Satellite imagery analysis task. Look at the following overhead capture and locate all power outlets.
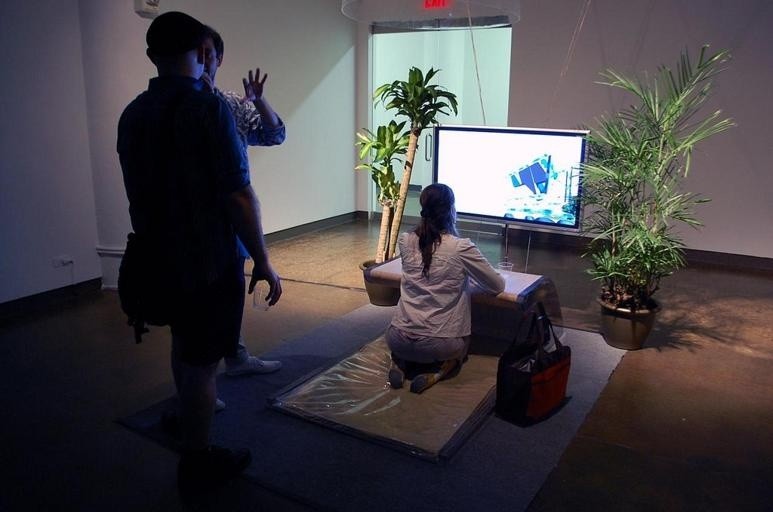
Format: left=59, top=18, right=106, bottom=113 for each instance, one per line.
left=52, top=254, right=72, bottom=267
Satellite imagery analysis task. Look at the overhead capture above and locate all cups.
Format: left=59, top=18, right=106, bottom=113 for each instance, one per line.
left=495, top=261, right=512, bottom=285
left=251, top=277, right=276, bottom=312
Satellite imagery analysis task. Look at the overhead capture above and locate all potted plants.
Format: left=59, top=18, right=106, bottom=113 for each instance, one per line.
left=578, top=42, right=741, bottom=351
left=359, top=63, right=460, bottom=306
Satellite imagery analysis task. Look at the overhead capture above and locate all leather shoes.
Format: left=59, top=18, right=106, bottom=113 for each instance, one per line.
left=388, top=351, right=406, bottom=389
left=409, top=359, right=449, bottom=394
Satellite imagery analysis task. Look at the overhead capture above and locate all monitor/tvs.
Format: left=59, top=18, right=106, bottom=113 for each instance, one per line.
left=432, top=124, right=591, bottom=235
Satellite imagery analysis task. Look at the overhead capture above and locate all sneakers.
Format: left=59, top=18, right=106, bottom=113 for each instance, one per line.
left=225, top=355, right=282, bottom=377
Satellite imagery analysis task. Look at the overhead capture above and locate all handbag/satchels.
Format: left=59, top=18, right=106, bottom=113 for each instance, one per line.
left=496, top=299, right=571, bottom=426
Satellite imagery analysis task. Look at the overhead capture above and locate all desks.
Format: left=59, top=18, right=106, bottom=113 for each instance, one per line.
left=366, top=252, right=563, bottom=356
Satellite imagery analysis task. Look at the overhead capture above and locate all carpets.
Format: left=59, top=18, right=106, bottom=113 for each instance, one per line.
left=108, top=291, right=626, bottom=512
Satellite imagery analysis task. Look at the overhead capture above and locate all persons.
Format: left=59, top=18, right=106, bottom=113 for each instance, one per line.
left=194, top=19, right=285, bottom=413
left=114, top=11, right=283, bottom=496
left=386, top=183, right=504, bottom=395
